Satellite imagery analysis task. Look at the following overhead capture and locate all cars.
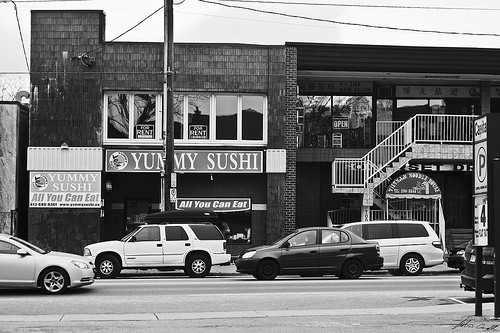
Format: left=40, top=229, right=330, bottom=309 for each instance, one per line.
left=0, top=232, right=95, bottom=295
left=235, top=226, right=385, bottom=281
left=447, top=241, right=469, bottom=273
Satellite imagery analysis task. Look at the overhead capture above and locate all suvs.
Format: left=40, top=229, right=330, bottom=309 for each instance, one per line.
left=83, top=222, right=232, bottom=278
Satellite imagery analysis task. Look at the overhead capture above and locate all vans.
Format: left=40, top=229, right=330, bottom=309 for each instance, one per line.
left=459, top=238, right=494, bottom=295
left=322, top=220, right=447, bottom=277
